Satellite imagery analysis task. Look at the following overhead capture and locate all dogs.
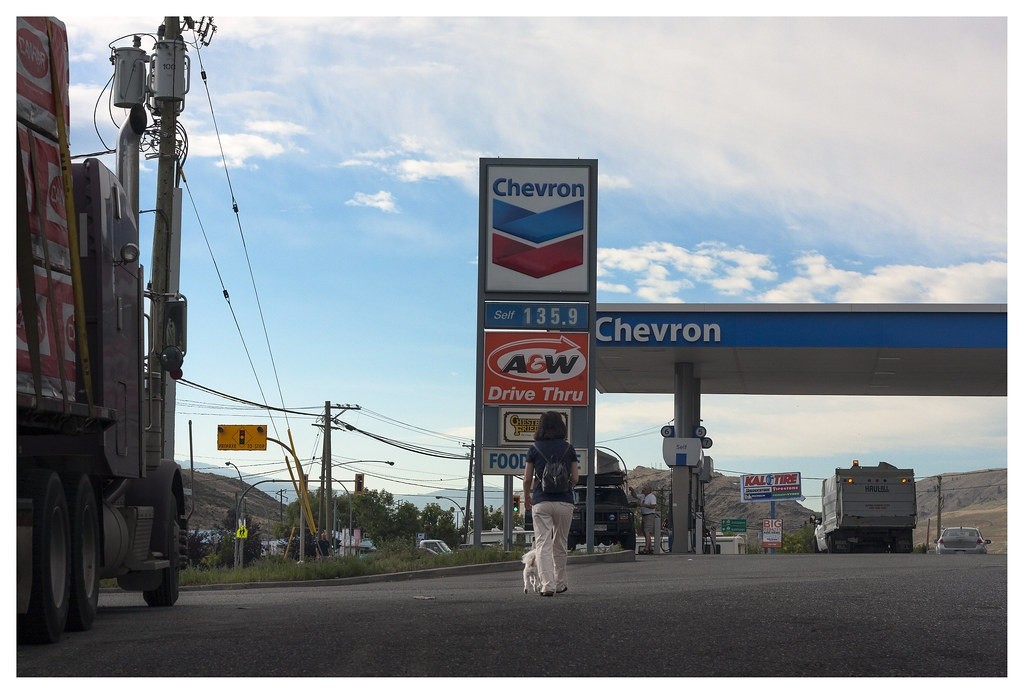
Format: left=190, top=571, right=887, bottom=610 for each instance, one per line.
left=521, top=540, right=543, bottom=595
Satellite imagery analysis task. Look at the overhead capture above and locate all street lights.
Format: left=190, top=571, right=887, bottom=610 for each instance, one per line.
left=435, top=495, right=466, bottom=519
left=222, top=460, right=247, bottom=510
left=318, top=473, right=353, bottom=537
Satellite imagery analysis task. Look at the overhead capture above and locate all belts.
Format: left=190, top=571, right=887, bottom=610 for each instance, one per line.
left=642, top=513, right=655, bottom=516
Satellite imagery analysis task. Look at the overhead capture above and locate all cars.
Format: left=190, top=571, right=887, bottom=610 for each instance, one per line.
left=420, top=539, right=451, bottom=553
left=935, top=525, right=991, bottom=555
left=566, top=484, right=639, bottom=553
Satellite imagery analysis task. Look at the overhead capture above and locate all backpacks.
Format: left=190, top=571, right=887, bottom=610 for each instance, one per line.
left=531, top=442, right=569, bottom=494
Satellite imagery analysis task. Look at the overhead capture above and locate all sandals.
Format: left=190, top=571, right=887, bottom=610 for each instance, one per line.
left=638, top=549, right=654, bottom=555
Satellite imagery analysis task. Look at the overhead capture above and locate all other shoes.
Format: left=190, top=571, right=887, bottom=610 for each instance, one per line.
left=555, top=587, right=568, bottom=593
left=540, top=591, right=554, bottom=597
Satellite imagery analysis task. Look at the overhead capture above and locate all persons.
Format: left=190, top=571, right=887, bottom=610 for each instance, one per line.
left=316, top=533, right=332, bottom=558
left=630, top=486, right=656, bottom=555
left=524, top=411, right=578, bottom=597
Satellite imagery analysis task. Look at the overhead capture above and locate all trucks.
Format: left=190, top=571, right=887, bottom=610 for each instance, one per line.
left=16, top=17, right=184, bottom=639
left=810, top=459, right=918, bottom=555
